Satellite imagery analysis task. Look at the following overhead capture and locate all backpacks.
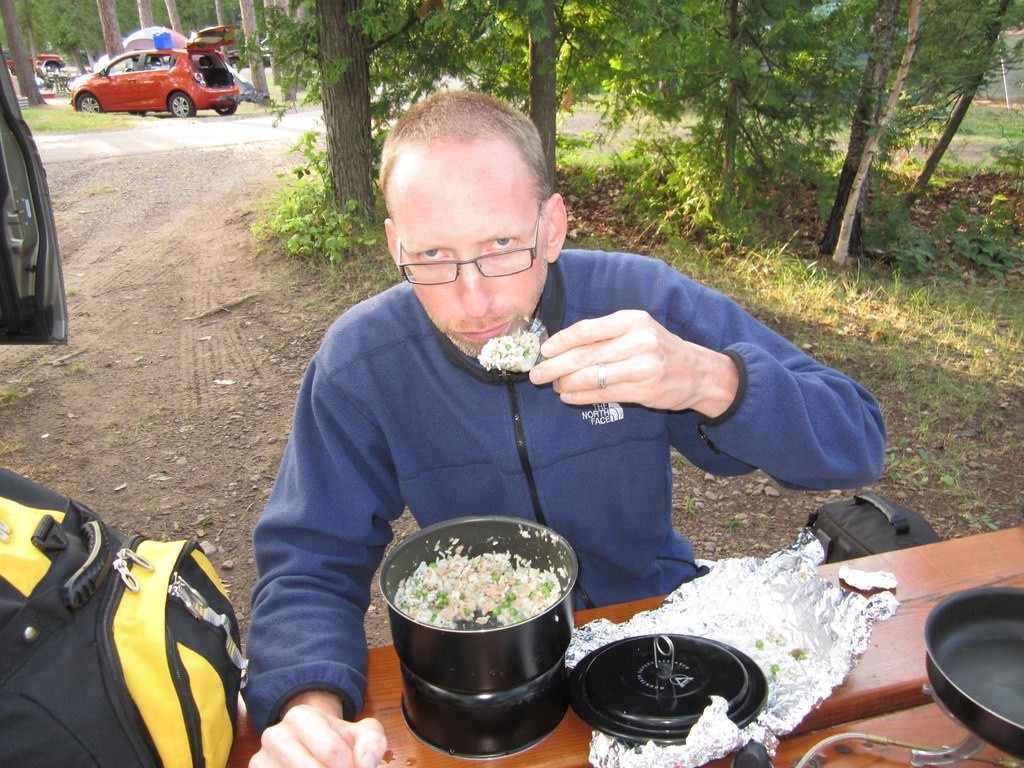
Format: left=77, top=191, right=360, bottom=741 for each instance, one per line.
left=2, top=469, right=242, bottom=765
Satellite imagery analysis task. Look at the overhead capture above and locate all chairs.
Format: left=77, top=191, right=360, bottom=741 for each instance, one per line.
left=198, top=57, right=210, bottom=69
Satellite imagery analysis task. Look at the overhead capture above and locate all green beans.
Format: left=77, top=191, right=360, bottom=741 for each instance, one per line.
left=399, top=561, right=555, bottom=627
left=498, top=333, right=530, bottom=357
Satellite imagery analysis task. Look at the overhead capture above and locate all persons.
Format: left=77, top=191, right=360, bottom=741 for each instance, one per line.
left=35, top=61, right=54, bottom=90
left=239, top=91, right=884, bottom=768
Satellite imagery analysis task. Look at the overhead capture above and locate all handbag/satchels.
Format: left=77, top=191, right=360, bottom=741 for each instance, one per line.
left=807, top=492, right=939, bottom=558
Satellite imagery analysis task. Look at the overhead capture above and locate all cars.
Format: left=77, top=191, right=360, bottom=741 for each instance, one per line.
left=70, top=23, right=242, bottom=121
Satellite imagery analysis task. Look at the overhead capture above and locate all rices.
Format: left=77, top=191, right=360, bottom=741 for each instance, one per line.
left=477, top=333, right=541, bottom=372
left=392, top=526, right=567, bottom=630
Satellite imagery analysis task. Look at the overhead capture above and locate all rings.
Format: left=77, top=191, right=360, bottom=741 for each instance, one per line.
left=598, top=362, right=608, bottom=389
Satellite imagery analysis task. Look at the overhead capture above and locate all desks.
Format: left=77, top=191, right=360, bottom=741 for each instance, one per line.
left=226, top=526, right=1024, bottom=768
left=47, top=75, right=72, bottom=98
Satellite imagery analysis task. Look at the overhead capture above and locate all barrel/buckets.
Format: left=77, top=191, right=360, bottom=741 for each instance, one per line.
left=153, top=31, right=172, bottom=50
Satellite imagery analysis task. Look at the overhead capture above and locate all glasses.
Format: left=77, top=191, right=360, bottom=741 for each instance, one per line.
left=397, top=206, right=542, bottom=285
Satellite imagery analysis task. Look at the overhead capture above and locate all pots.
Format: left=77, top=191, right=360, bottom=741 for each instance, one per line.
left=924, top=587, right=1024, bottom=759
left=378, top=514, right=578, bottom=761
